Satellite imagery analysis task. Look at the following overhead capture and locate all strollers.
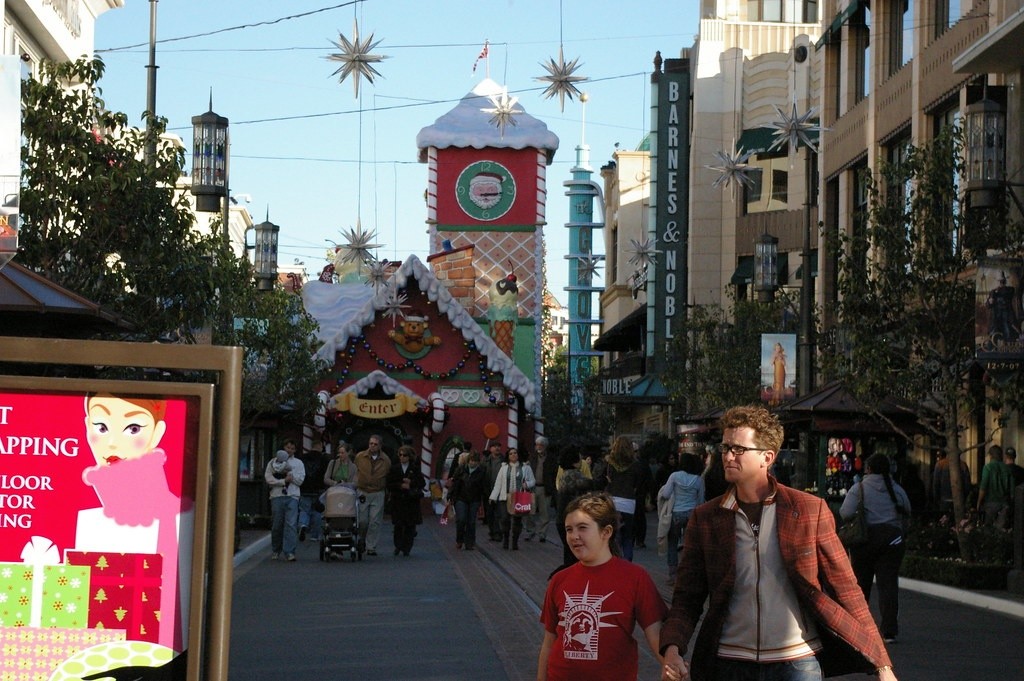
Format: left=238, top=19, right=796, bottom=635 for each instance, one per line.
left=318, top=486, right=362, bottom=563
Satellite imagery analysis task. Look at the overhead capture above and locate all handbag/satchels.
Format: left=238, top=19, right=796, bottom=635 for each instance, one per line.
left=853, top=523, right=906, bottom=565
left=409, top=486, right=425, bottom=500
left=506, top=486, right=538, bottom=517
left=440, top=505, right=456, bottom=526
left=315, top=491, right=326, bottom=513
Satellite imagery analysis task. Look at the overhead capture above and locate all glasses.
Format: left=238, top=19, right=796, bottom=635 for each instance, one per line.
left=718, top=443, right=768, bottom=455
left=399, top=453, right=408, bottom=457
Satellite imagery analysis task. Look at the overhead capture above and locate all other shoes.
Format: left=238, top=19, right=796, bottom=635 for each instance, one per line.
left=394, top=547, right=411, bottom=556
left=456, top=541, right=475, bottom=549
left=884, top=634, right=897, bottom=643
left=298, top=524, right=320, bottom=541
left=362, top=547, right=377, bottom=556
left=638, top=542, right=647, bottom=547
left=666, top=576, right=677, bottom=585
left=272, top=552, right=297, bottom=561
left=490, top=536, right=547, bottom=551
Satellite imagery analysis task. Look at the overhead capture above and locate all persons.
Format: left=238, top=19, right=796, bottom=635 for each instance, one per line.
left=659, top=403, right=901, bottom=681
left=264, top=434, right=425, bottom=562
left=429, top=436, right=790, bottom=586
left=537, top=490, right=690, bottom=681
left=929, top=450, right=972, bottom=511
left=825, top=438, right=862, bottom=503
left=978, top=445, right=1024, bottom=560
left=838, top=455, right=911, bottom=644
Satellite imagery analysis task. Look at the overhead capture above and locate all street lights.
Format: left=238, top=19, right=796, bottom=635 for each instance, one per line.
left=753, top=226, right=815, bottom=471
left=143, top=83, right=229, bottom=262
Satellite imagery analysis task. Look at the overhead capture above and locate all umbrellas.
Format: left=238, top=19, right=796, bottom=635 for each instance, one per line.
left=775, top=372, right=947, bottom=456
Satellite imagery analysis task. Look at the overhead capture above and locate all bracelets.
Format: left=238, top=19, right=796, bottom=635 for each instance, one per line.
left=874, top=666, right=891, bottom=675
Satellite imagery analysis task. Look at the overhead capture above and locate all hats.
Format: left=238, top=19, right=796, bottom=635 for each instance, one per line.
left=277, top=449, right=289, bottom=462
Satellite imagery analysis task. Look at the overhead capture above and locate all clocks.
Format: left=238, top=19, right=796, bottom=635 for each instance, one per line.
left=455, top=161, right=517, bottom=222
